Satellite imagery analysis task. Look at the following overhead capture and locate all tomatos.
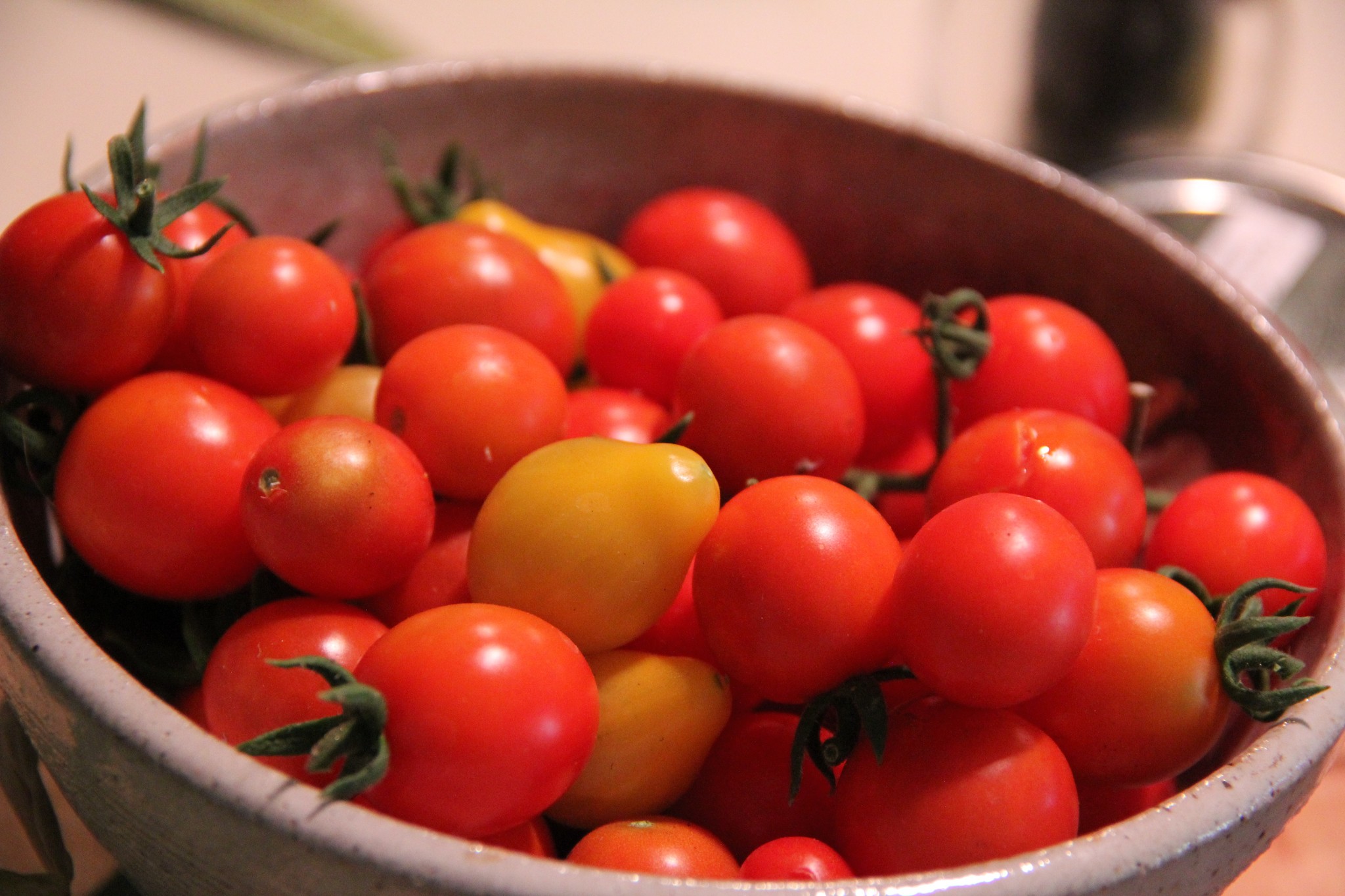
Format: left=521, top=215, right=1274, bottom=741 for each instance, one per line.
left=0, top=84, right=1331, bottom=886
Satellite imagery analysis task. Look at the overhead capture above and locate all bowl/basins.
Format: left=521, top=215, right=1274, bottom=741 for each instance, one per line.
left=0, top=55, right=1344, bottom=896
